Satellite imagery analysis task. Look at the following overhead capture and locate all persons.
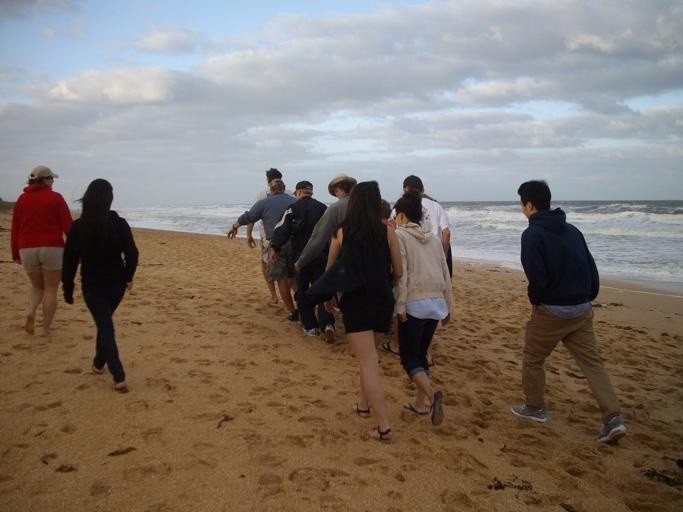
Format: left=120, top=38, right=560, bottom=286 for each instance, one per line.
left=61, top=179, right=139, bottom=393
left=294, top=181, right=403, bottom=443
left=229, top=169, right=358, bottom=342
left=512, top=179, right=628, bottom=443
left=382, top=174, right=451, bottom=424
left=11, top=165, right=74, bottom=336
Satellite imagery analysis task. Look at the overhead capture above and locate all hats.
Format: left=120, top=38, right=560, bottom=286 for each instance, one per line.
left=292, top=180, right=313, bottom=196
left=29, top=164, right=57, bottom=180
left=268, top=178, right=284, bottom=187
left=328, top=176, right=357, bottom=197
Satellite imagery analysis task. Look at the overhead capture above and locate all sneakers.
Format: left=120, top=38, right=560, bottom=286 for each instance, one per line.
left=289, top=309, right=337, bottom=343
left=511, top=404, right=547, bottom=423
left=597, top=416, right=626, bottom=443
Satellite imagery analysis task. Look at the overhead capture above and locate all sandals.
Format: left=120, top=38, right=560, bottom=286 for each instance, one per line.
left=353, top=402, right=371, bottom=418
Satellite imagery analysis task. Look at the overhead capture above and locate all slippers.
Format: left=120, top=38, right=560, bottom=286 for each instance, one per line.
left=431, top=390, right=445, bottom=425
left=381, top=339, right=399, bottom=355
left=368, top=424, right=394, bottom=444
left=404, top=401, right=430, bottom=416
